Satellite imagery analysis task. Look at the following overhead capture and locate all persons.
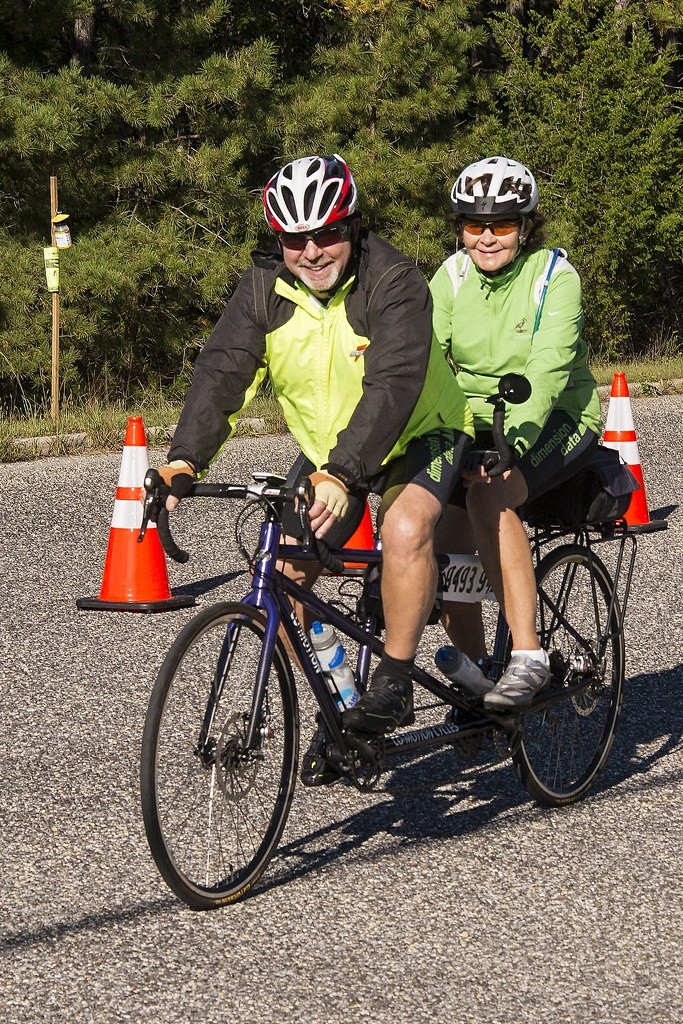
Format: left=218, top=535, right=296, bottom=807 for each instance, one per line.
left=141, top=154, right=476, bottom=787
left=427, top=157, right=603, bottom=750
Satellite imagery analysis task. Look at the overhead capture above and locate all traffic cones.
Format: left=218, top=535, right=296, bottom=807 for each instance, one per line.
left=76, top=415, right=196, bottom=614
left=326, top=500, right=376, bottom=575
left=592, top=371, right=669, bottom=533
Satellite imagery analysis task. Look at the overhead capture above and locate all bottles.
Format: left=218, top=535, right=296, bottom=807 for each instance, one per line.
left=310, top=621, right=361, bottom=713
left=434, top=646, right=493, bottom=695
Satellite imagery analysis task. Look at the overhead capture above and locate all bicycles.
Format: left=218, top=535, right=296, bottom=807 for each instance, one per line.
left=136, top=372, right=640, bottom=912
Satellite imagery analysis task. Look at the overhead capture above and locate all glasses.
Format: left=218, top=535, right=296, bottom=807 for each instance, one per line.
left=460, top=218, right=521, bottom=236
left=273, top=225, right=347, bottom=250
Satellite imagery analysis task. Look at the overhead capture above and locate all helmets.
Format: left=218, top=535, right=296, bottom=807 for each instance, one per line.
left=449, top=156, right=539, bottom=214
left=263, top=153, right=357, bottom=233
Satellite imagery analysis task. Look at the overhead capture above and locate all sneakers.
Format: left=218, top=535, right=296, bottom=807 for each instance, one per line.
left=483, top=649, right=554, bottom=708
left=342, top=670, right=416, bottom=732
left=300, top=711, right=346, bottom=787
